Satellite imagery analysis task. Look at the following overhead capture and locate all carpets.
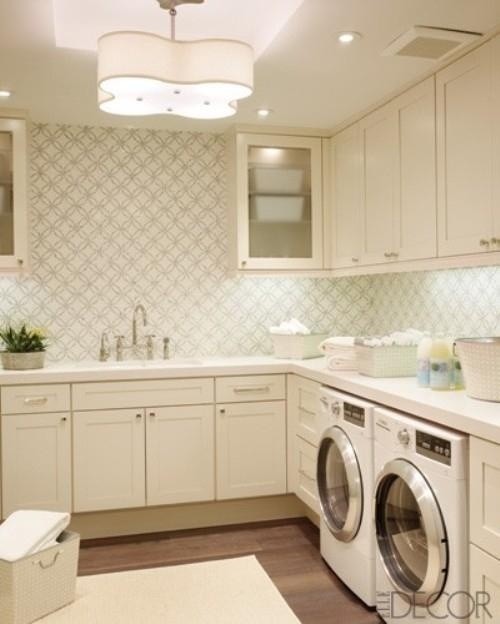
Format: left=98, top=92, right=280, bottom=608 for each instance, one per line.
left=32, top=554, right=302, bottom=624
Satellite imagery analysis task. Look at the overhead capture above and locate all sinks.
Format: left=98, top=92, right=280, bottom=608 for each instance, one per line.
left=86, top=357, right=205, bottom=370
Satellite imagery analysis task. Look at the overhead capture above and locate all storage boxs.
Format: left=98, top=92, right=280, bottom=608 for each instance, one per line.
left=0, top=509, right=81, bottom=624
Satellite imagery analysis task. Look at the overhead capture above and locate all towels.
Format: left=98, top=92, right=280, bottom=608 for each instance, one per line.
left=320, top=337, right=355, bottom=354
left=327, top=355, right=358, bottom=371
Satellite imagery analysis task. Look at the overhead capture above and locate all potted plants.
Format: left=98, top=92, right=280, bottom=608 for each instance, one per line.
left=0, top=321, right=53, bottom=369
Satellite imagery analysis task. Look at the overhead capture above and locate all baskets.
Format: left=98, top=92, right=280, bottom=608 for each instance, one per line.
left=319, top=336, right=354, bottom=369
left=272, top=333, right=326, bottom=358
left=456, top=338, right=499, bottom=402
left=355, top=337, right=415, bottom=378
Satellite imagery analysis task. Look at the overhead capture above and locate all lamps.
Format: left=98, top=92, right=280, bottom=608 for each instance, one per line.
left=95, top=0, right=254, bottom=120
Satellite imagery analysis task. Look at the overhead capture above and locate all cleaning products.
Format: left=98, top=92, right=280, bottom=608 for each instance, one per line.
left=418, top=326, right=468, bottom=392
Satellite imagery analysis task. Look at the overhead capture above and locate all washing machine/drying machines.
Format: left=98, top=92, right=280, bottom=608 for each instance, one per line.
left=373, top=406, right=470, bottom=624
left=315, top=386, right=382, bottom=608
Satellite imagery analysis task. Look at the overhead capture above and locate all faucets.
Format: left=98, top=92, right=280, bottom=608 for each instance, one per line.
left=133, top=303, right=148, bottom=345
left=100, top=332, right=111, bottom=361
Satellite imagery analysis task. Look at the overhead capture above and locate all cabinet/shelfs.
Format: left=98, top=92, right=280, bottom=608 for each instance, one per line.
left=0, top=110, right=28, bottom=275
left=361, top=73, right=436, bottom=276
left=0, top=382, right=72, bottom=522
left=228, top=123, right=322, bottom=276
left=436, top=34, right=500, bottom=269
left=288, top=374, right=322, bottom=517
left=466, top=435, right=500, bottom=624
left=214, top=374, right=287, bottom=502
left=330, top=96, right=364, bottom=277
left=72, top=377, right=217, bottom=513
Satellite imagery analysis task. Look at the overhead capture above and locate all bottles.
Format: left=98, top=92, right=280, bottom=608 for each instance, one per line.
left=416, top=331, right=465, bottom=391
